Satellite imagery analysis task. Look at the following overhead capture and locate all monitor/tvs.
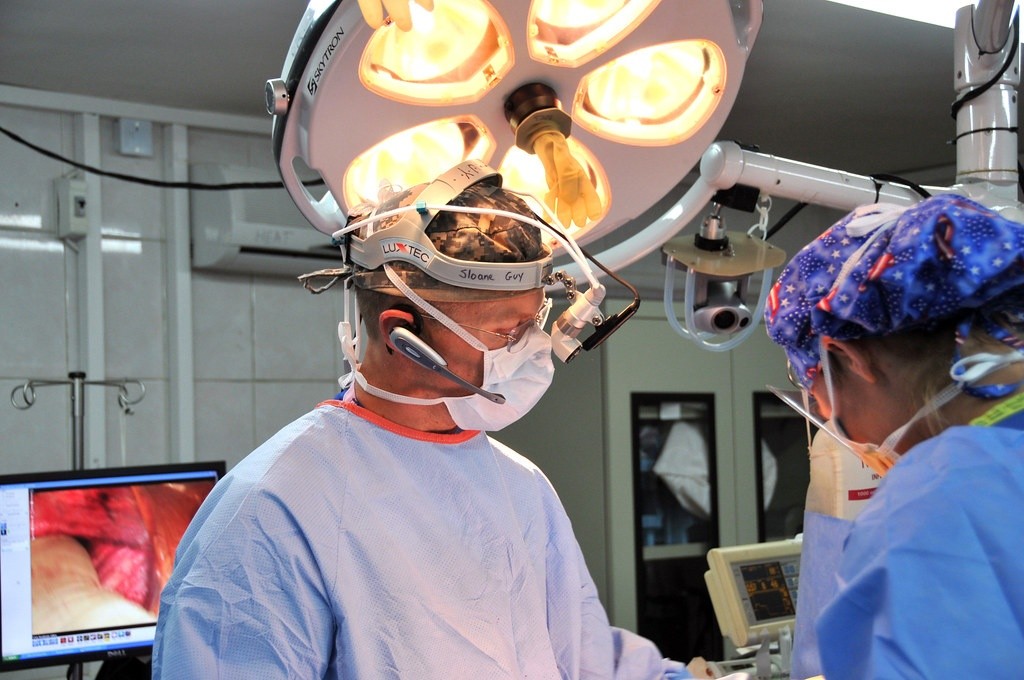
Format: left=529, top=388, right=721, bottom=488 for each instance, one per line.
left=0, top=460, right=229, bottom=676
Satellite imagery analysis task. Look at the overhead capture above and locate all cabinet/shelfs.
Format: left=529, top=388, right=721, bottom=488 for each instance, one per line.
left=599, top=299, right=819, bottom=664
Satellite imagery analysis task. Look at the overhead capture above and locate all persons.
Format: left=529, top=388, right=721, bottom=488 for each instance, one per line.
left=763, top=192, right=1024, bottom=680
left=150, top=161, right=721, bottom=680
left=30, top=534, right=156, bottom=635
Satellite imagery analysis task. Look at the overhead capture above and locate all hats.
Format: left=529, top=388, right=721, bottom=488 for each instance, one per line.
left=815, top=193, right=1024, bottom=346
left=765, top=204, right=908, bottom=380
left=343, top=180, right=547, bottom=302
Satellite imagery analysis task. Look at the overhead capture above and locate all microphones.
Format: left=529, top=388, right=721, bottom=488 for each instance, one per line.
left=389, top=324, right=506, bottom=411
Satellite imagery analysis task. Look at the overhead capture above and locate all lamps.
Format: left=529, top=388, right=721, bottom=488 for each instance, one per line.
left=264, top=0, right=764, bottom=294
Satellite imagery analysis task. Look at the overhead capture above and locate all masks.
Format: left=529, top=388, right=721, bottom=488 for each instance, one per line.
left=413, top=296, right=555, bottom=431
left=806, top=353, right=939, bottom=522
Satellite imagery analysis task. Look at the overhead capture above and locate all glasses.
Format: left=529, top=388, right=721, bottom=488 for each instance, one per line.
left=783, top=358, right=826, bottom=390
left=421, top=298, right=554, bottom=355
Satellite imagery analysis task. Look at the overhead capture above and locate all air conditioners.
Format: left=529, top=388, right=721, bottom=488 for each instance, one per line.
left=187, top=159, right=347, bottom=281
